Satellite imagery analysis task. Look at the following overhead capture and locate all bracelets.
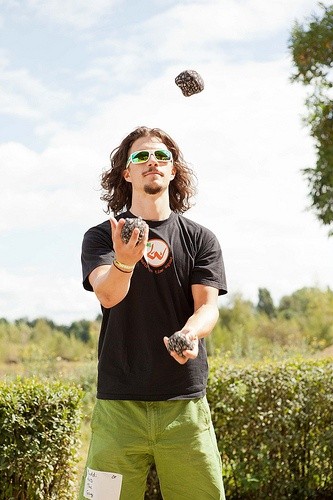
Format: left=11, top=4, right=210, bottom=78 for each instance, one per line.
left=112, top=259, right=136, bottom=274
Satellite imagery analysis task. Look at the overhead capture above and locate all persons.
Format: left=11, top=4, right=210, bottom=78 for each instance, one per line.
left=77, top=127, right=228, bottom=500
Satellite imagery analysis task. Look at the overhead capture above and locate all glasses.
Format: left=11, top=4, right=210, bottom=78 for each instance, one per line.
left=126, top=150, right=173, bottom=168
